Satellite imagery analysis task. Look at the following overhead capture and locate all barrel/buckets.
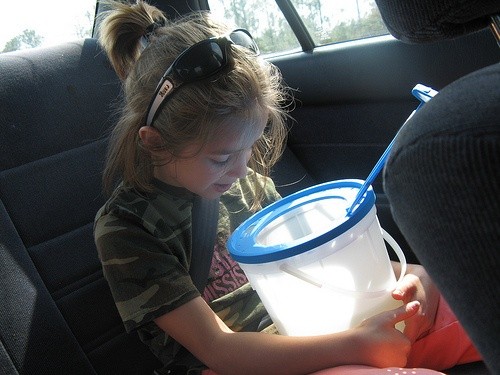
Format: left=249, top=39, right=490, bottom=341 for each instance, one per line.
left=226, top=180, right=408, bottom=338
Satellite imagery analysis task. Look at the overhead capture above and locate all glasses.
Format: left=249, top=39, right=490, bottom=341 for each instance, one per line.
left=145, top=28, right=260, bottom=127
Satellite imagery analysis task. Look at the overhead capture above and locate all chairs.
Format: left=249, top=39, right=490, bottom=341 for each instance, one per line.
left=0, top=39, right=190, bottom=375
left=375, top=0, right=500, bottom=375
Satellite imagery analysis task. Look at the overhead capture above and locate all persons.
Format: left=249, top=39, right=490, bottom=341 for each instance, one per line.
left=90, top=1, right=482, bottom=374
